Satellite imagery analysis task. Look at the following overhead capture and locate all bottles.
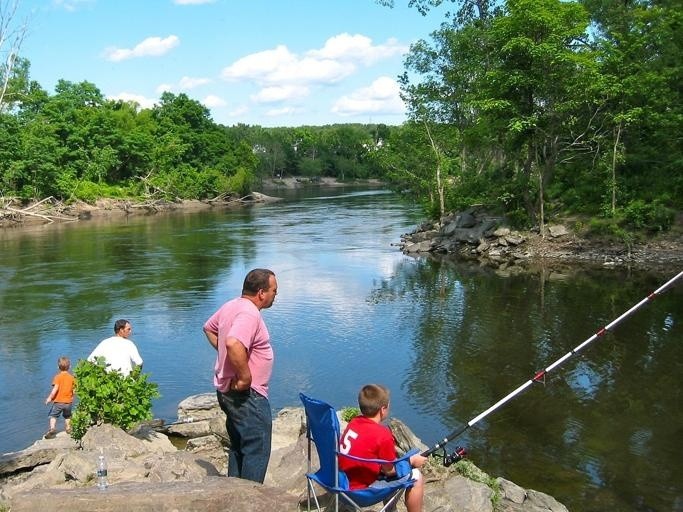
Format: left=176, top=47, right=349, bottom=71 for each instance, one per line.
left=97, top=455, right=109, bottom=493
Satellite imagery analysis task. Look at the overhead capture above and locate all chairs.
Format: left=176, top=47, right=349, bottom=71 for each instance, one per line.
left=299, top=393, right=417, bottom=512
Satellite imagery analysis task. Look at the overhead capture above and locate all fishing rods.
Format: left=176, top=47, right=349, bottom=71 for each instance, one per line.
left=420, top=270, right=683, bottom=468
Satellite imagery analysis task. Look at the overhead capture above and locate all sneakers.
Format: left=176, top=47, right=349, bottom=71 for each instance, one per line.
left=45, top=429, right=57, bottom=439
left=64, top=425, right=72, bottom=432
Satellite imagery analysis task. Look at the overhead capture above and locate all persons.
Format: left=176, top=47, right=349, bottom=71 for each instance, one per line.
left=87, top=319, right=143, bottom=379
left=203, top=268, right=277, bottom=484
left=45, top=356, right=76, bottom=439
left=338, top=384, right=428, bottom=512
